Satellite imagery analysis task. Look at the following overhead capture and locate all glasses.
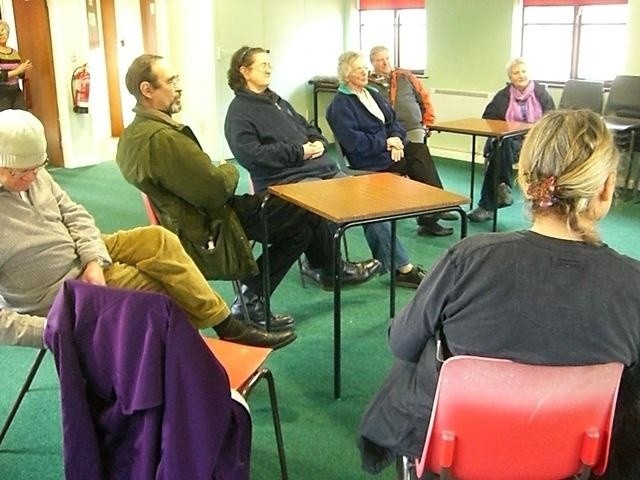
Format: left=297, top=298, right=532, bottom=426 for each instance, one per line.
left=6, top=159, right=50, bottom=178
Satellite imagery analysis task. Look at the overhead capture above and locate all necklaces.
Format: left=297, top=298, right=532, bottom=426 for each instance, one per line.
left=0, top=47, right=12, bottom=58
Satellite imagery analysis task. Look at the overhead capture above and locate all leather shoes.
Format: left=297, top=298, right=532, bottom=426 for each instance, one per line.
left=416, top=209, right=458, bottom=239
left=219, top=326, right=297, bottom=351
left=229, top=293, right=296, bottom=325
left=299, top=258, right=384, bottom=295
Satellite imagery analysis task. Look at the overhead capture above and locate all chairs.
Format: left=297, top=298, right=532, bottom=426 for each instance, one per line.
left=555, top=78, right=604, bottom=120
left=56, top=276, right=294, bottom=480
left=140, top=186, right=251, bottom=326
left=391, top=355, right=626, bottom=480
left=247, top=173, right=350, bottom=292
left=484, top=80, right=548, bottom=174
left=323, top=101, right=409, bottom=178
left=594, top=76, right=640, bottom=205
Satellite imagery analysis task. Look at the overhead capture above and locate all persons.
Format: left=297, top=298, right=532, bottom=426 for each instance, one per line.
left=1, top=20, right=36, bottom=112
left=223, top=44, right=428, bottom=289
left=325, top=50, right=460, bottom=237
left=0, top=108, right=298, bottom=351
left=465, top=57, right=557, bottom=223
left=355, top=103, right=640, bottom=480
left=365, top=45, right=437, bottom=146
left=115, top=53, right=384, bottom=328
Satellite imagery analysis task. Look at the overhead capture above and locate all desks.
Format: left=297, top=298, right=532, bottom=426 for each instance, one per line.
left=417, top=115, right=540, bottom=239
left=308, top=70, right=388, bottom=138
left=248, top=168, right=477, bottom=404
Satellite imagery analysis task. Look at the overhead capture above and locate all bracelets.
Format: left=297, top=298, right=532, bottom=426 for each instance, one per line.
left=96, top=256, right=105, bottom=267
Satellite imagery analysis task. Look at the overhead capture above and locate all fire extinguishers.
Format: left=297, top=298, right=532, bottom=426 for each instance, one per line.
left=71, top=63, right=92, bottom=115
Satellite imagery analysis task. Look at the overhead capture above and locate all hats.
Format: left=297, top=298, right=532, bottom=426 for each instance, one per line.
left=0, top=108, right=48, bottom=170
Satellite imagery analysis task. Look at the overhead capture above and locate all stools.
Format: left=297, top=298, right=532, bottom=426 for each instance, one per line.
left=0, top=341, right=50, bottom=456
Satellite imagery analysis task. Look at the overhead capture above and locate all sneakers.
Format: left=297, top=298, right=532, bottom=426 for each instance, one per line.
left=496, top=183, right=514, bottom=208
left=392, top=266, right=433, bottom=289
left=466, top=206, right=494, bottom=222
